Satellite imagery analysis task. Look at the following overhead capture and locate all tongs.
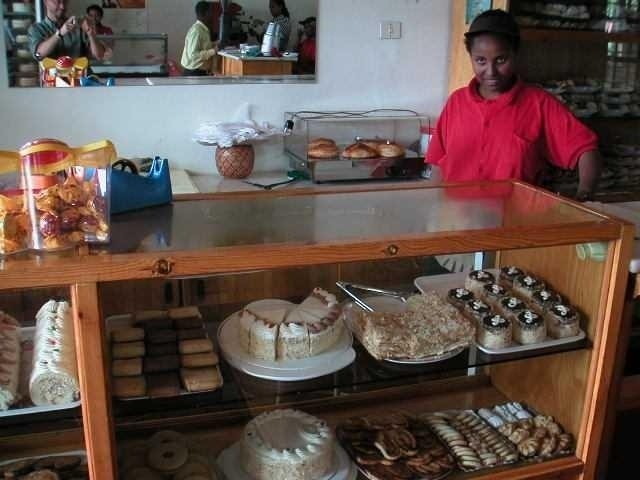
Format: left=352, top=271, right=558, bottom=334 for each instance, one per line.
left=335, top=278, right=411, bottom=313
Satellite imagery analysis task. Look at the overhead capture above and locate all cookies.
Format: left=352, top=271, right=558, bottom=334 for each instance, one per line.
left=338, top=401, right=574, bottom=480
left=0, top=174, right=109, bottom=256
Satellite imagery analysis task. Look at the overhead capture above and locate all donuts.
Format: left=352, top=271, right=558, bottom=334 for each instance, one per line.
left=118, top=431, right=216, bottom=478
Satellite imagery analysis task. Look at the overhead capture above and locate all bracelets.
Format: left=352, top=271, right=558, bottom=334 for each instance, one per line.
left=55, top=27, right=65, bottom=39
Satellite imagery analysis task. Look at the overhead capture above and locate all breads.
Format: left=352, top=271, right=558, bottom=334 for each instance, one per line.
left=110, top=307, right=220, bottom=395
left=0, top=264, right=79, bottom=480
left=307, top=138, right=405, bottom=159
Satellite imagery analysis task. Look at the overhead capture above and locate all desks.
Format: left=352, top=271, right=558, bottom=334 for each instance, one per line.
left=216, top=46, right=299, bottom=76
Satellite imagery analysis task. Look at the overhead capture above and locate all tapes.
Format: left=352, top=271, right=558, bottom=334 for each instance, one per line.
left=87, top=74, right=107, bottom=83
left=112, top=158, right=153, bottom=175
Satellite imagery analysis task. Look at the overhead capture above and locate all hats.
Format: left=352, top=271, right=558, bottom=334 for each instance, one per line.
left=298, top=17, right=315, bottom=25
left=463, top=10, right=521, bottom=43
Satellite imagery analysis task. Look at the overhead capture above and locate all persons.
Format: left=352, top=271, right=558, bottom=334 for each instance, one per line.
left=295, top=16, right=317, bottom=74
left=86, top=4, right=115, bottom=60
left=246, top=0, right=291, bottom=49
left=180, top=0, right=232, bottom=77
left=424, top=7, right=601, bottom=203
left=26, top=0, right=106, bottom=65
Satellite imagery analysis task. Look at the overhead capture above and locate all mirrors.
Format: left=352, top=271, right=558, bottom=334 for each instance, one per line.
left=1, top=0, right=317, bottom=88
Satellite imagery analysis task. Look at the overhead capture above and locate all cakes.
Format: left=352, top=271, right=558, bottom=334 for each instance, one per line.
left=238, top=288, right=342, bottom=360
left=241, top=409, right=335, bottom=478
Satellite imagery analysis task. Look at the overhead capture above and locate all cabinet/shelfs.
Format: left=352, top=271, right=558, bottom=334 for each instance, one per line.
left=1, top=178, right=635, bottom=480
left=444, top=0, right=639, bottom=204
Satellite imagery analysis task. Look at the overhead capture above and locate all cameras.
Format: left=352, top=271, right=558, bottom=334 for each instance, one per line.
left=71, top=16, right=85, bottom=26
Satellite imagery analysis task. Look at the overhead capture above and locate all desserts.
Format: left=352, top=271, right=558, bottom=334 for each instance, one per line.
left=349, top=291, right=474, bottom=360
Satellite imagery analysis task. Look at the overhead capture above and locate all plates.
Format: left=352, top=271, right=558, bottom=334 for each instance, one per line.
left=216, top=296, right=356, bottom=384
left=215, top=433, right=359, bottom=480
left=343, top=293, right=472, bottom=366
